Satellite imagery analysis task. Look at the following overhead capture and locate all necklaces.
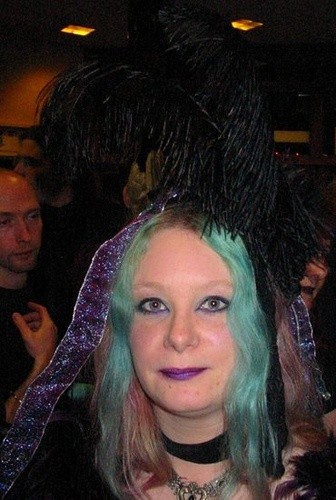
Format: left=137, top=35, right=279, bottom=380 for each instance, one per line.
left=160, top=428, right=229, bottom=464
left=170, top=470, right=232, bottom=500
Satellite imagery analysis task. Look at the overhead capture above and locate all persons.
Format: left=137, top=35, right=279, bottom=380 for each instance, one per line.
left=13, top=126, right=120, bottom=277
left=0, top=168, right=78, bottom=439
left=300, top=241, right=336, bottom=436
left=0, top=192, right=336, bottom=500
